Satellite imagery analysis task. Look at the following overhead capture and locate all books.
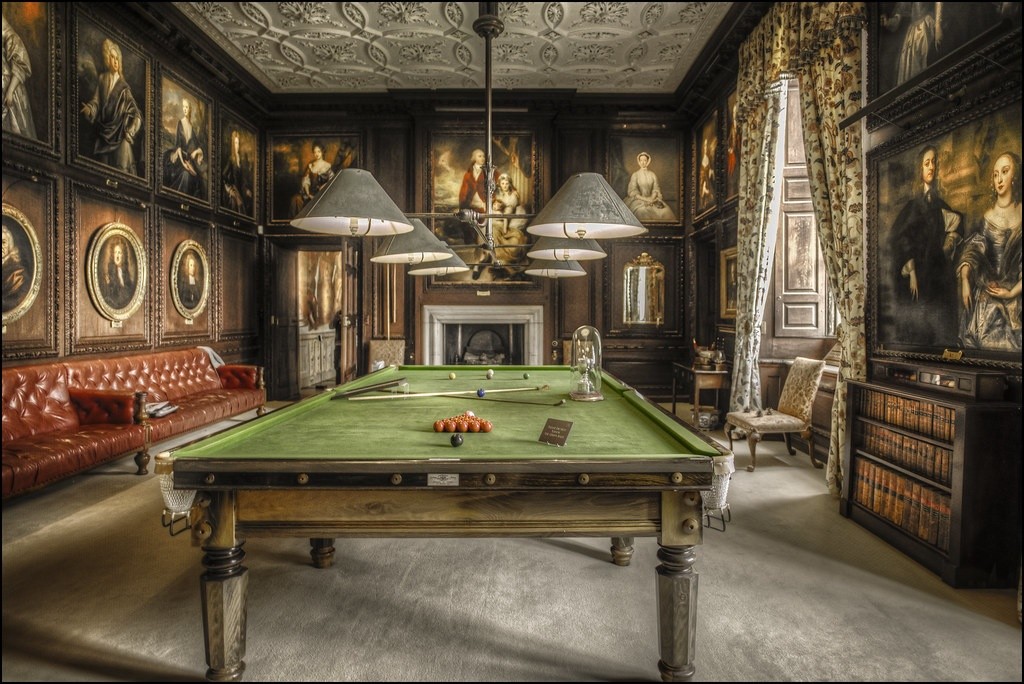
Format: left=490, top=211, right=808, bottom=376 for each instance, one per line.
left=852, top=387, right=956, bottom=555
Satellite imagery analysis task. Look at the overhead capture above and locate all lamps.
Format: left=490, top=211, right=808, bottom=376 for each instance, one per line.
left=290, top=7, right=649, bottom=278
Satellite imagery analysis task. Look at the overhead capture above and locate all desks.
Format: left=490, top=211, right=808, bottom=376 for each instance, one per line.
left=154, top=363, right=735, bottom=682
left=672, top=361, right=729, bottom=427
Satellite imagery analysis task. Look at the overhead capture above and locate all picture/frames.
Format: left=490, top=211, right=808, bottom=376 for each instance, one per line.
left=720, top=247, right=737, bottom=318
left=866, top=90, right=1023, bottom=375
left=0, top=0, right=265, bottom=362
left=601, top=236, right=689, bottom=340
left=265, top=128, right=364, bottom=228
left=691, top=79, right=742, bottom=226
left=423, top=129, right=544, bottom=292
left=604, top=128, right=685, bottom=228
left=866, top=2, right=1022, bottom=134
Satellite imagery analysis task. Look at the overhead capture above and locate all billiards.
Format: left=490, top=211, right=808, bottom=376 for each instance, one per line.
left=486, top=369, right=494, bottom=379
left=433, top=411, right=492, bottom=449
left=523, top=373, right=529, bottom=379
left=477, top=388, right=485, bottom=397
left=448, top=372, right=457, bottom=380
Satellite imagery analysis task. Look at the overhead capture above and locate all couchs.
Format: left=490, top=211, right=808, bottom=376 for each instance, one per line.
left=2, top=346, right=266, bottom=504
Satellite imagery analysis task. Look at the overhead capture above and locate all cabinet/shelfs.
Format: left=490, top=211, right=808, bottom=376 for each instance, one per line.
left=840, top=379, right=1024, bottom=588
left=299, top=329, right=336, bottom=387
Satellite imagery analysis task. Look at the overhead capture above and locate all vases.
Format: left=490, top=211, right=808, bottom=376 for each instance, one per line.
left=689, top=407, right=720, bottom=431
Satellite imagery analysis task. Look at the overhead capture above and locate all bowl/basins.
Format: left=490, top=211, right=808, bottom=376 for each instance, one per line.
left=690, top=408, right=721, bottom=432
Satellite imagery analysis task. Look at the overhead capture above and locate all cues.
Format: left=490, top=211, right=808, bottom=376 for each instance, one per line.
left=347, top=388, right=539, bottom=401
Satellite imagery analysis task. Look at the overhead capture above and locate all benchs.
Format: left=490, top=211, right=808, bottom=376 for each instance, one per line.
left=724, top=356, right=826, bottom=472
